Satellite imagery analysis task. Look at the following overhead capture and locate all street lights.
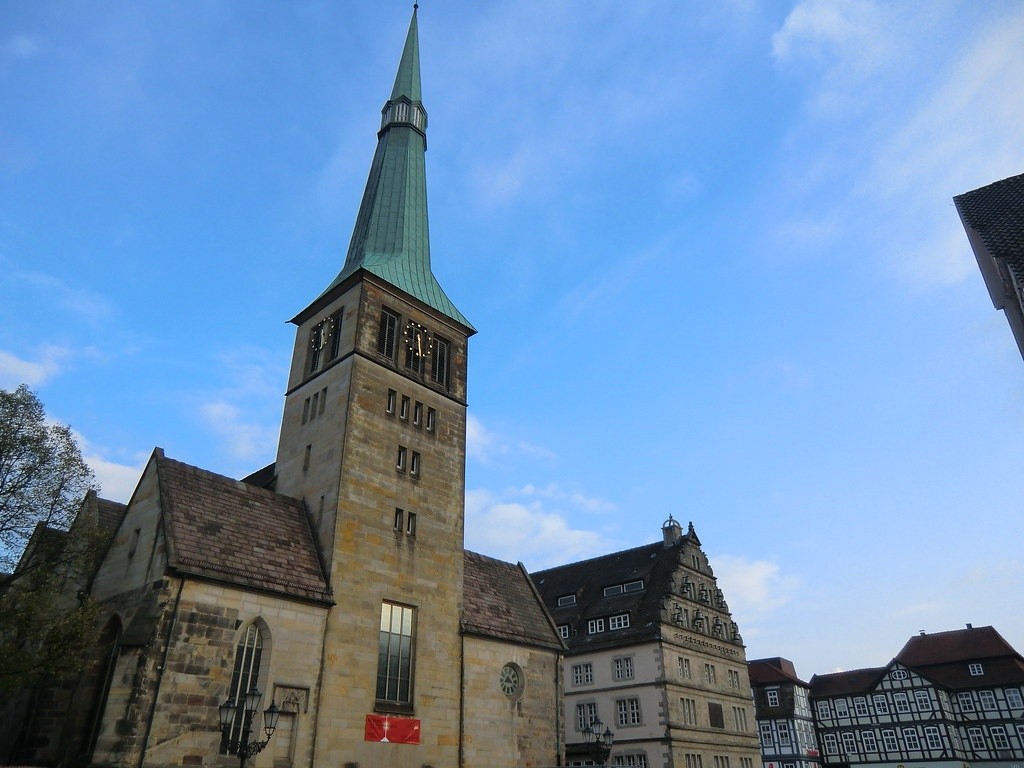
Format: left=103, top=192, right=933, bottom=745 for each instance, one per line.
left=218, top=682, right=281, bottom=767
left=581, top=718, right=614, bottom=767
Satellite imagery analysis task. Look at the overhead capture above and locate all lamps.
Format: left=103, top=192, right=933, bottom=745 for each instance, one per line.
left=219, top=682, right=280, bottom=768
left=582, top=716, right=614, bottom=766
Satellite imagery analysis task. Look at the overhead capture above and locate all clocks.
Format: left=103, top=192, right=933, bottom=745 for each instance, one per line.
left=312, top=317, right=335, bottom=352
left=404, top=322, right=432, bottom=358
